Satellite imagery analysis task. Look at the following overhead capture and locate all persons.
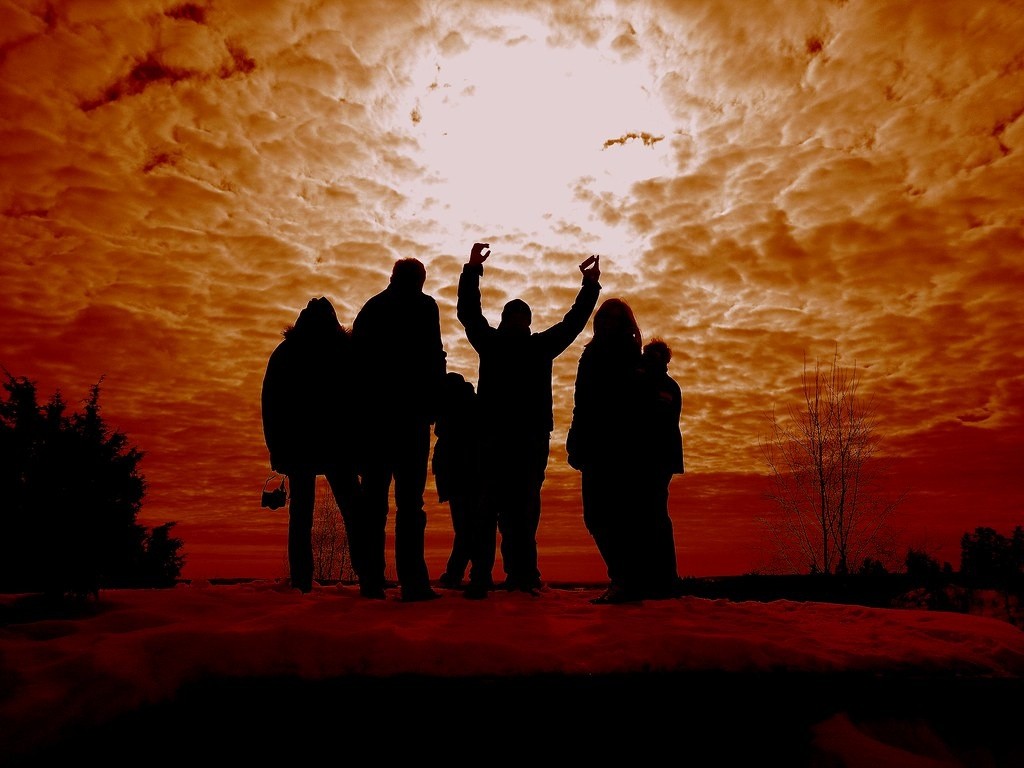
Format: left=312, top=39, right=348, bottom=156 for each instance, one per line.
left=261, top=244, right=601, bottom=601
left=567, top=299, right=684, bottom=603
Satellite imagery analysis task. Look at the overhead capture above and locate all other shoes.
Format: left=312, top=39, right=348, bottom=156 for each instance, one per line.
left=588, top=578, right=642, bottom=604
left=642, top=579, right=680, bottom=600
left=496, top=574, right=542, bottom=592
left=360, top=583, right=387, bottom=600
left=460, top=579, right=496, bottom=592
left=271, top=578, right=313, bottom=593
left=435, top=571, right=462, bottom=589
left=401, top=583, right=444, bottom=603
left=463, top=583, right=488, bottom=600
